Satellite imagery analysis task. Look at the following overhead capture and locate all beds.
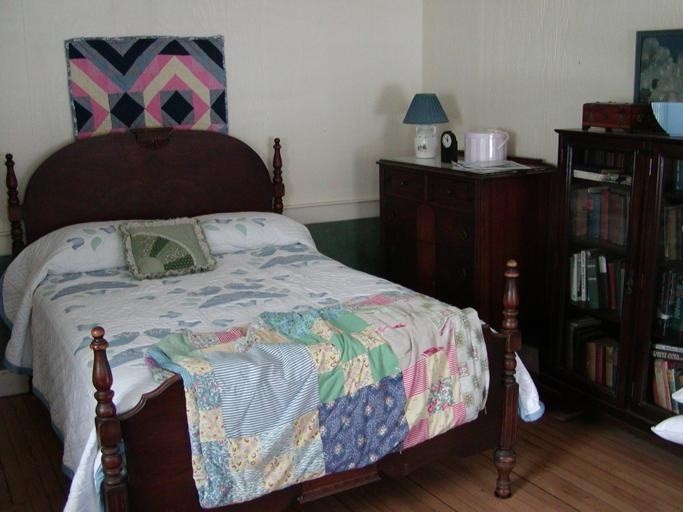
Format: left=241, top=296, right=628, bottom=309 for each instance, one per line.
left=0, top=128, right=546, bottom=512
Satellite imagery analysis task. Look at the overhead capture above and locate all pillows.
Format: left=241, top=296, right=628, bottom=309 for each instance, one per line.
left=118, top=217, right=217, bottom=280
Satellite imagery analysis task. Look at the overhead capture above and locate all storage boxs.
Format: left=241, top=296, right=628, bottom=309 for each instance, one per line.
left=580, top=102, right=634, bottom=134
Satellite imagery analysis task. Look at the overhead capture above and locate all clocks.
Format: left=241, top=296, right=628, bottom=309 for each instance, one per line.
left=441, top=133, right=456, bottom=160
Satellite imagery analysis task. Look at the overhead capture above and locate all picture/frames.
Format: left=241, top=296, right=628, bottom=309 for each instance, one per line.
left=634, top=30, right=683, bottom=108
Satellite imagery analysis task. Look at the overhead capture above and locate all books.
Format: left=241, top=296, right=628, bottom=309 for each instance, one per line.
left=561, top=147, right=683, bottom=414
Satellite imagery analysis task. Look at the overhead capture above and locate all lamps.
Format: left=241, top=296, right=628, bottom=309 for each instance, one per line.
left=402, top=92, right=451, bottom=157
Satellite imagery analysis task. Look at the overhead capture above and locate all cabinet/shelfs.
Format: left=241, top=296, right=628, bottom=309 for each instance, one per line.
left=553, top=125, right=682, bottom=455
left=372, top=152, right=558, bottom=414
